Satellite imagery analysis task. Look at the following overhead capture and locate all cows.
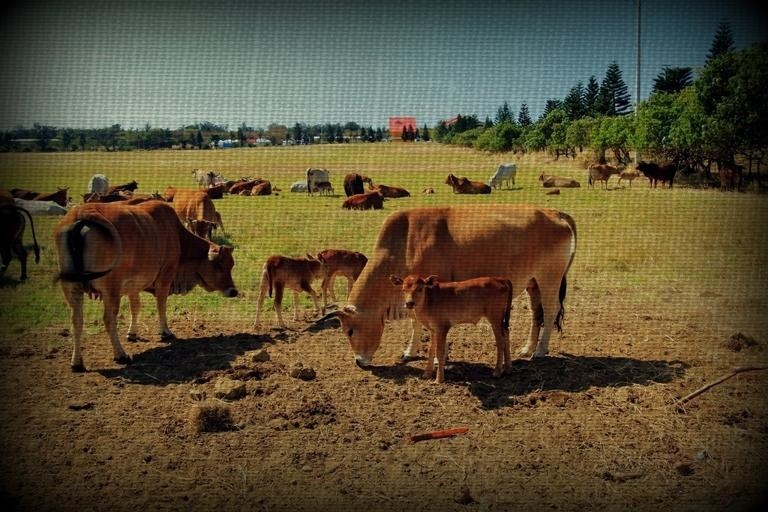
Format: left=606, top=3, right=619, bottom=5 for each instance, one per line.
left=253, top=249, right=368, bottom=329
left=81, top=169, right=271, bottom=238
left=52, top=199, right=239, bottom=373
left=444, top=162, right=522, bottom=195
left=0, top=203, right=40, bottom=287
left=13, top=186, right=69, bottom=206
left=540, top=152, right=679, bottom=189
left=304, top=159, right=411, bottom=209
left=301, top=196, right=577, bottom=383
